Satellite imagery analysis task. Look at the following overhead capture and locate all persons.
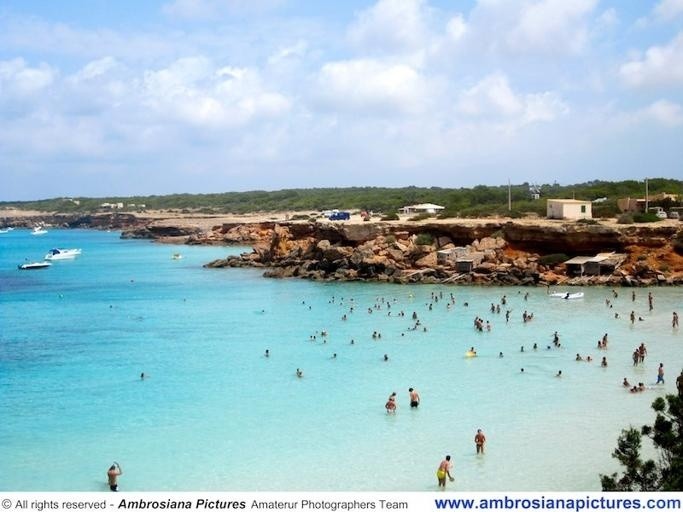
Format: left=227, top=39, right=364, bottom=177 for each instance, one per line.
left=474, top=428, right=487, bottom=454
left=107, top=461, right=123, bottom=492
left=435, top=452, right=454, bottom=488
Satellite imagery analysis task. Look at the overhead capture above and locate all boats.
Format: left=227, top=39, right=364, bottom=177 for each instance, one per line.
left=18, top=261, right=52, bottom=269
left=45, top=248, right=82, bottom=259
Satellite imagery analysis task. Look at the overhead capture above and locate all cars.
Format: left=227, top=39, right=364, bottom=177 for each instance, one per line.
left=324, top=210, right=350, bottom=221
